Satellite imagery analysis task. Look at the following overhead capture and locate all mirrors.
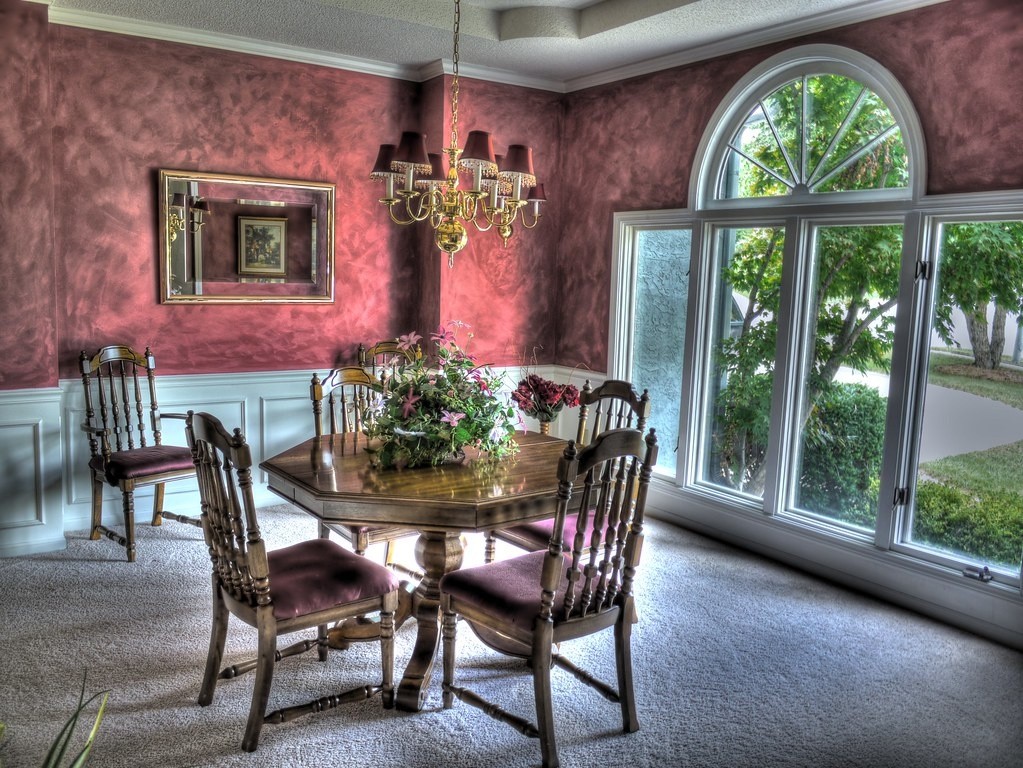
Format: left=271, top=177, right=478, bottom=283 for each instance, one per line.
left=157, top=167, right=337, bottom=305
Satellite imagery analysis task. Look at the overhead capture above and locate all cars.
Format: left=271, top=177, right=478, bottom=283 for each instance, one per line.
left=730, top=295, right=746, bottom=345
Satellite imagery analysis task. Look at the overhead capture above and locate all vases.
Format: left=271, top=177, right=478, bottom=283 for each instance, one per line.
left=379, top=426, right=466, bottom=469
left=540, top=422, right=550, bottom=436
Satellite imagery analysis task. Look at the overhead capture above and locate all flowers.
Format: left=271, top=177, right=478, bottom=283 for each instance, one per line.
left=511, top=372, right=582, bottom=416
left=366, top=321, right=528, bottom=457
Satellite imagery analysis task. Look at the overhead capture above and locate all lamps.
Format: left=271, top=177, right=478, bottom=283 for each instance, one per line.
left=368, top=0, right=550, bottom=268
left=167, top=192, right=211, bottom=243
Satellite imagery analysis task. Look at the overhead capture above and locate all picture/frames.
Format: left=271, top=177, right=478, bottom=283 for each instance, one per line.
left=236, top=214, right=290, bottom=279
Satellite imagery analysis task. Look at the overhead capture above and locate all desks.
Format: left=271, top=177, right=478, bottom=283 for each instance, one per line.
left=257, top=427, right=642, bottom=715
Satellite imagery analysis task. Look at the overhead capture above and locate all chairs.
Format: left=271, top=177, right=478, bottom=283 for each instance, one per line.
left=357, top=340, right=423, bottom=434
left=437, top=427, right=661, bottom=768
left=311, top=365, right=421, bottom=560
left=78, top=343, right=208, bottom=564
left=485, top=377, right=652, bottom=624
left=182, top=409, right=401, bottom=752
